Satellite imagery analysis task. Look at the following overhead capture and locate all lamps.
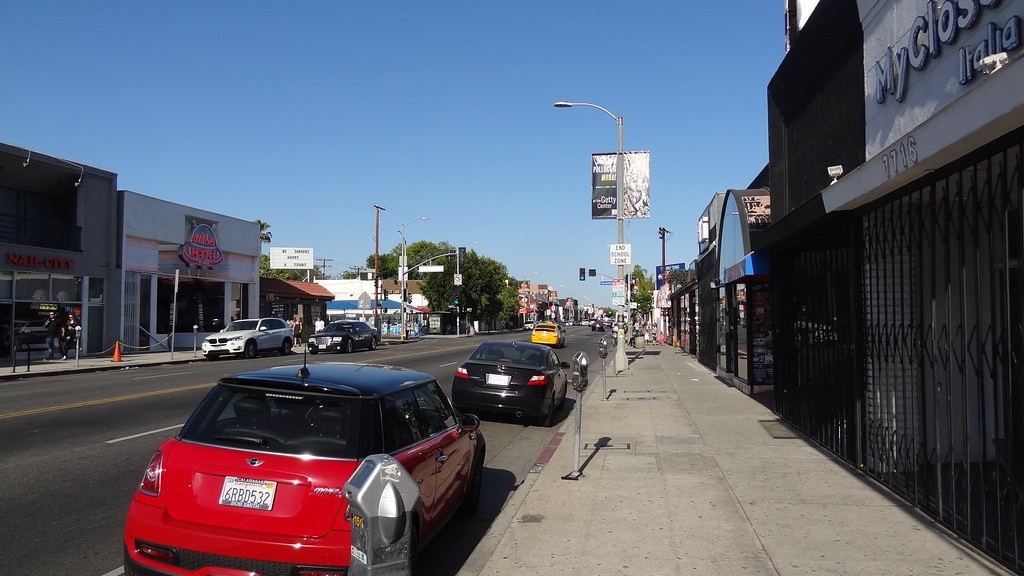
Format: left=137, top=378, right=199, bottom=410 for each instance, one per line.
left=827, top=165, right=843, bottom=185
left=980, top=52, right=1007, bottom=74
left=22, top=146, right=31, bottom=167
left=57, top=157, right=83, bottom=186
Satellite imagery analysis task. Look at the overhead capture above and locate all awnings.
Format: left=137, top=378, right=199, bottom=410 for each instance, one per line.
left=519, top=307, right=543, bottom=313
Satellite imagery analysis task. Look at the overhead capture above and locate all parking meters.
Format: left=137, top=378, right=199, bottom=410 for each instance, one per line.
left=342, top=453, right=420, bottom=576
left=612, top=332, right=618, bottom=377
left=75, top=326, right=82, bottom=368
left=192, top=325, right=198, bottom=357
left=599, top=338, right=608, bottom=401
left=572, top=350, right=589, bottom=477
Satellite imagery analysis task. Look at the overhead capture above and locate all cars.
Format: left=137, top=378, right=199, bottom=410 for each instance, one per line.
left=307, top=321, right=379, bottom=354
left=122, top=341, right=487, bottom=576
left=201, top=317, right=294, bottom=360
left=19, top=320, right=48, bottom=333
left=530, top=322, right=566, bottom=348
left=451, top=340, right=570, bottom=427
left=523, top=317, right=616, bottom=332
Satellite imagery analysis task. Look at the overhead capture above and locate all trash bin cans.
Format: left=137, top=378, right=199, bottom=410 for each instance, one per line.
left=634, top=331, right=645, bottom=349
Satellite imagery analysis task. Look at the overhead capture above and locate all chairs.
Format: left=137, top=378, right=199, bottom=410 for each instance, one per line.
left=528, top=353, right=539, bottom=360
left=234, top=396, right=270, bottom=425
left=485, top=349, right=504, bottom=360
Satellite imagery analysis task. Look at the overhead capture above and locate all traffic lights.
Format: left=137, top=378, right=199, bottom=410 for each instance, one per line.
left=580, top=268, right=585, bottom=280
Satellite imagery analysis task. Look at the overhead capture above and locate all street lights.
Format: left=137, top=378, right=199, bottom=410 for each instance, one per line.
left=555, top=101, right=629, bottom=372
left=396, top=216, right=429, bottom=341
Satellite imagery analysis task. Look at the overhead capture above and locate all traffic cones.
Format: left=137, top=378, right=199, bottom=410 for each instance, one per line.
left=110, top=341, right=125, bottom=362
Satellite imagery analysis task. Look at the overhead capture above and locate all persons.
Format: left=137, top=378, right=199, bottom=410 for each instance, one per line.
left=61, top=312, right=81, bottom=360
left=315, top=316, right=325, bottom=334
left=681, top=330, right=690, bottom=345
left=406, top=321, right=410, bottom=340
left=633, top=321, right=639, bottom=334
left=628, top=330, right=639, bottom=346
left=286, top=318, right=294, bottom=328
left=57, top=291, right=71, bottom=312
left=292, top=316, right=302, bottom=347
left=43, top=310, right=65, bottom=362
